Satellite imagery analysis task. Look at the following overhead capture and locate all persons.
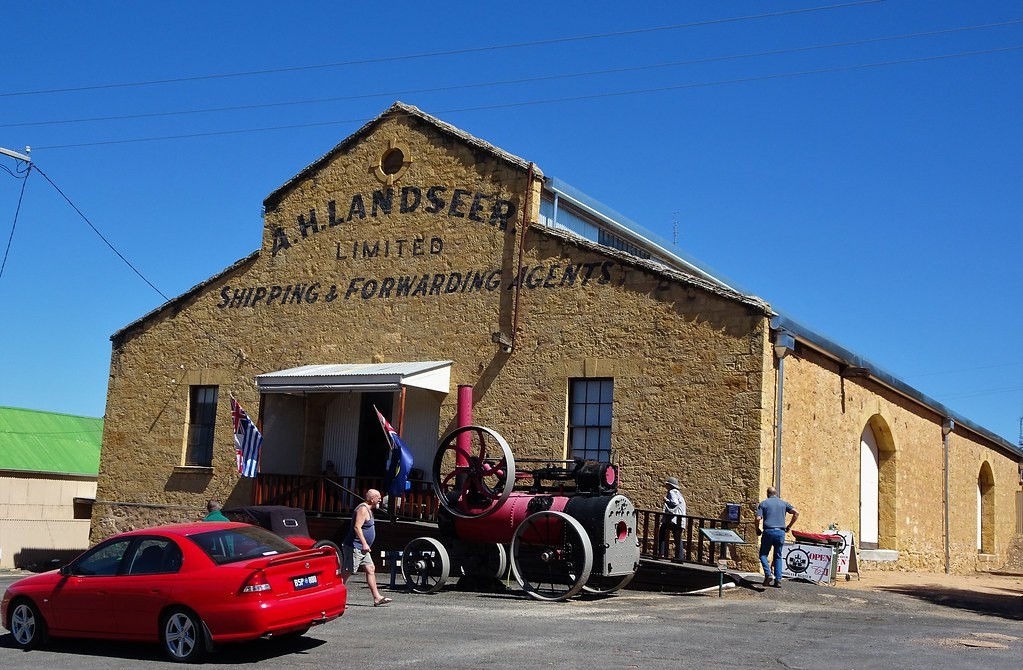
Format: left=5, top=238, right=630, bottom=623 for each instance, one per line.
left=649, top=477, right=686, bottom=564
left=203, top=500, right=235, bottom=557
left=341, top=489, right=392, bottom=609
left=755, top=487, right=800, bottom=587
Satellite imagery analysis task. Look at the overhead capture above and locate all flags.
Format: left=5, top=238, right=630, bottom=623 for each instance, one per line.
left=230, top=397, right=262, bottom=478
left=375, top=408, right=414, bottom=496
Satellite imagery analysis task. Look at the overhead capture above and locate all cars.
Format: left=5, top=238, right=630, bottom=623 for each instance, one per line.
left=0, top=520, right=348, bottom=665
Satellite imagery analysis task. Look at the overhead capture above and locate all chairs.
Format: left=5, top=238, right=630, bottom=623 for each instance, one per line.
left=162, top=543, right=177, bottom=564
left=138, top=545, right=164, bottom=574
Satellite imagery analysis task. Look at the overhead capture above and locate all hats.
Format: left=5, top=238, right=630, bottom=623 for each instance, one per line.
left=664, top=477, right=680, bottom=489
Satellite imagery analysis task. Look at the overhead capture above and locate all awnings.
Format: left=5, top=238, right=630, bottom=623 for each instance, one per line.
left=255, top=361, right=451, bottom=394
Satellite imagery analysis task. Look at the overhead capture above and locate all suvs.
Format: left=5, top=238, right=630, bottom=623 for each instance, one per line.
left=218, top=507, right=344, bottom=578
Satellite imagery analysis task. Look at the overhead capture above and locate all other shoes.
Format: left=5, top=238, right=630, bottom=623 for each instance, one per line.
left=672, top=559, right=683, bottom=564
left=763, top=576, right=773, bottom=586
left=658, top=553, right=664, bottom=557
left=774, top=582, right=781, bottom=588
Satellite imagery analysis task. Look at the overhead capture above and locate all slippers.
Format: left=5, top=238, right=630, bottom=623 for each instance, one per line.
left=374, top=597, right=393, bottom=606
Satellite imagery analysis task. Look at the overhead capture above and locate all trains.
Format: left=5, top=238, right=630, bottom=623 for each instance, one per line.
left=399, top=382, right=640, bottom=602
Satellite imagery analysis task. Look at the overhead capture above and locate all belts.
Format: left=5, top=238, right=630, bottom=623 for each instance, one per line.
left=764, top=527, right=785, bottom=531
